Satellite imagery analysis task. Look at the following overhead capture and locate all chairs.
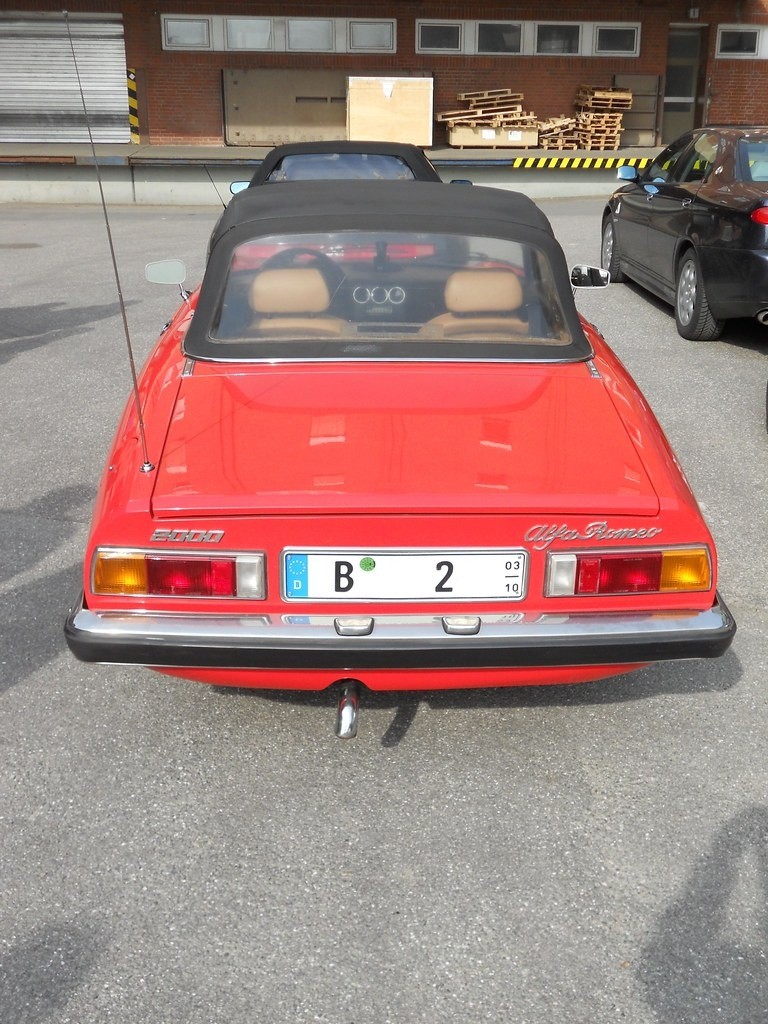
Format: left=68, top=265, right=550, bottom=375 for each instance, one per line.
left=240, top=267, right=353, bottom=338
left=414, top=268, right=533, bottom=339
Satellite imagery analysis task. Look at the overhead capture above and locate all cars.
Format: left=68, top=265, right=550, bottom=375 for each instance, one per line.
left=600, top=127, right=768, bottom=341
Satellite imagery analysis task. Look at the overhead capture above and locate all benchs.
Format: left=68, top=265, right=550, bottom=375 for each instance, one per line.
left=446, top=123, right=538, bottom=150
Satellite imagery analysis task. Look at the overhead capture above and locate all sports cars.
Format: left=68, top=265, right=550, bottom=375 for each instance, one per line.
left=226, top=138, right=475, bottom=268
left=63, top=171, right=737, bottom=739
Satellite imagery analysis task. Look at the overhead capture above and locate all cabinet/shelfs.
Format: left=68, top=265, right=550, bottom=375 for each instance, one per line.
left=346, top=77, right=434, bottom=147
